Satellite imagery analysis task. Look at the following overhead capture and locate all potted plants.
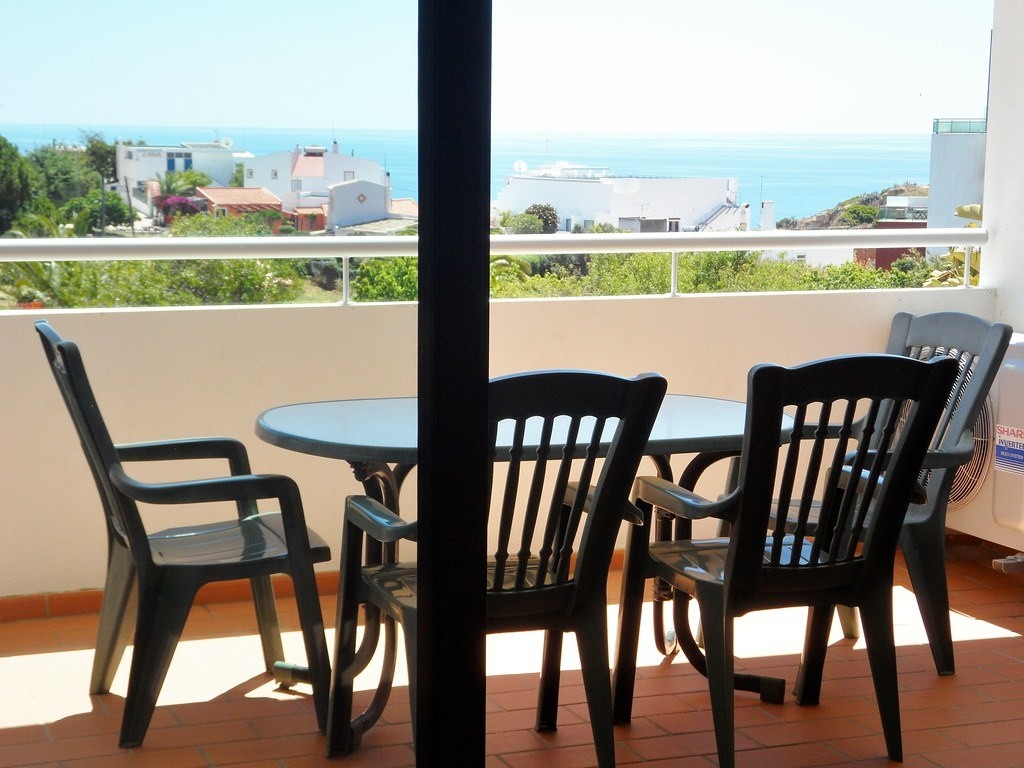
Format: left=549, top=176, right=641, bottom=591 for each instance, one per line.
left=17, top=288, right=47, bottom=309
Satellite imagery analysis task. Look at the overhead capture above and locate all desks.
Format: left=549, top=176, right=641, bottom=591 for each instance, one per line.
left=254, top=396, right=803, bottom=751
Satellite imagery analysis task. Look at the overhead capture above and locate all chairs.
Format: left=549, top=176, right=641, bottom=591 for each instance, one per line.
left=719, top=311, right=1013, bottom=676
left=609, top=353, right=960, bottom=768
left=324, top=368, right=668, bottom=768
left=34, top=317, right=334, bottom=748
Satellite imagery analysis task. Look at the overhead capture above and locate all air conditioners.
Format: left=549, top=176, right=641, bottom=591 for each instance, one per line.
left=889, top=332, right=1024, bottom=573
